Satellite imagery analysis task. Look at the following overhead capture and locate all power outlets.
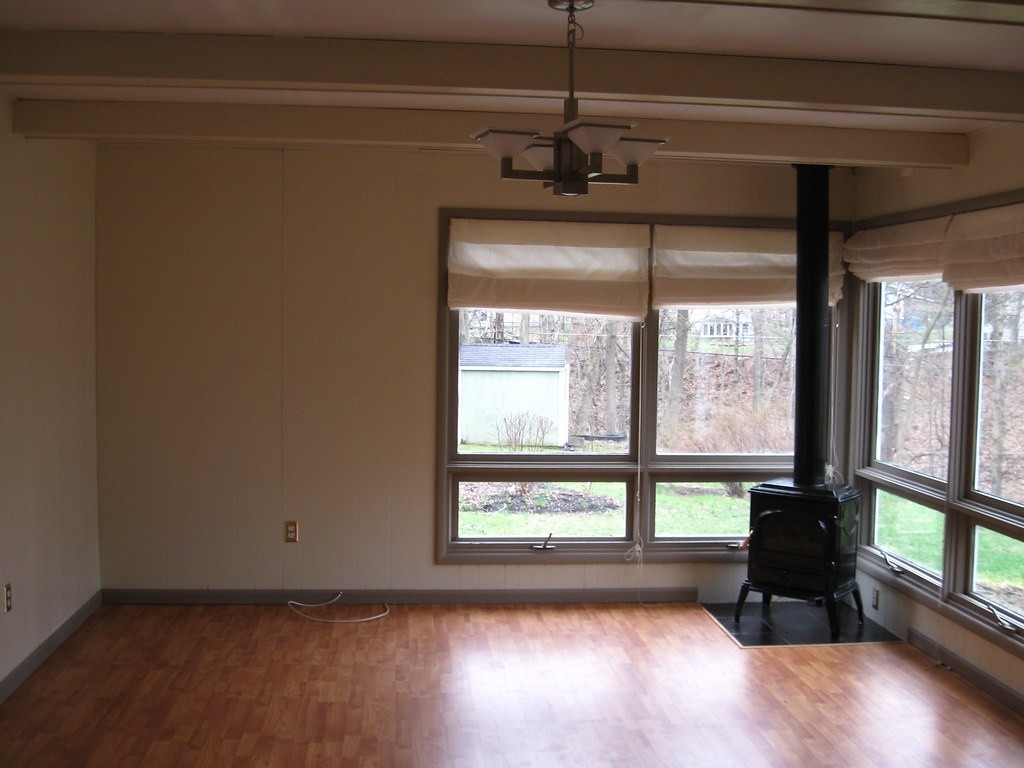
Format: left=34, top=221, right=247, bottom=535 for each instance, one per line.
left=285, top=521, right=298, bottom=543
left=5, top=583, right=12, bottom=613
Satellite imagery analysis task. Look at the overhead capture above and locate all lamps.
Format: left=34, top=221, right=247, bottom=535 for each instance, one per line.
left=468, top=1, right=670, bottom=195
